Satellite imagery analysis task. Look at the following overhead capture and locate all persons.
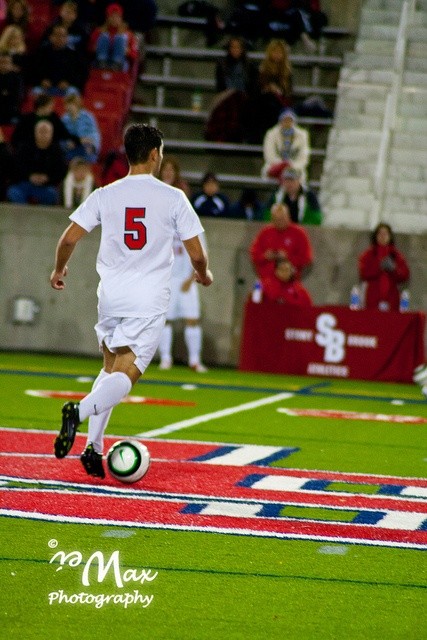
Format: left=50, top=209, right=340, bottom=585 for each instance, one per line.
left=31, top=25, right=81, bottom=98
left=230, top=188, right=261, bottom=219
left=50, top=124, right=213, bottom=479
left=200, top=0, right=327, bottom=51
left=247, top=39, right=293, bottom=145
left=0, top=0, right=29, bottom=46
left=358, top=223, right=408, bottom=311
left=62, top=156, right=93, bottom=208
left=203, top=37, right=257, bottom=143
left=7, top=119, right=64, bottom=206
left=38, top=1, right=88, bottom=63
left=157, top=231, right=208, bottom=374
left=156, top=156, right=186, bottom=196
left=248, top=259, right=312, bottom=306
left=0, top=50, right=27, bottom=126
left=88, top=3, right=137, bottom=74
left=0, top=25, right=35, bottom=89
left=12, top=96, right=79, bottom=161
left=61, top=95, right=100, bottom=164
left=190, top=171, right=229, bottom=218
left=260, top=109, right=309, bottom=183
left=263, top=167, right=321, bottom=226
left=249, top=204, right=312, bottom=282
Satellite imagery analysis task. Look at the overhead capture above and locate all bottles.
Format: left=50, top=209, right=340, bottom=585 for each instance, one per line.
left=399, top=289, right=410, bottom=314
left=350, top=284, right=361, bottom=311
left=253, top=280, right=263, bottom=303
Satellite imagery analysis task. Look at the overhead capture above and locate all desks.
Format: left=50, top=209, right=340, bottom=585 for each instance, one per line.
left=239, top=302, right=426, bottom=383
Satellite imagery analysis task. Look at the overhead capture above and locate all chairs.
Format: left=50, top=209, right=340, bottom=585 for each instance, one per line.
left=85, top=70, right=128, bottom=147
left=28, top=2, right=51, bottom=32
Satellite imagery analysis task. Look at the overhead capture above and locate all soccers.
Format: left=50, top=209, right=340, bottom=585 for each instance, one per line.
left=107, top=437, right=150, bottom=484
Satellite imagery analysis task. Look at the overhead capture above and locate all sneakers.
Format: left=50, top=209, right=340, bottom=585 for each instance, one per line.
left=80, top=444, right=106, bottom=479
left=53, top=401, right=81, bottom=458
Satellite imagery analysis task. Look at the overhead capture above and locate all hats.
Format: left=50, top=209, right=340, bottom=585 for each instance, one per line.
left=279, top=108, right=297, bottom=120
left=107, top=4, right=122, bottom=17
